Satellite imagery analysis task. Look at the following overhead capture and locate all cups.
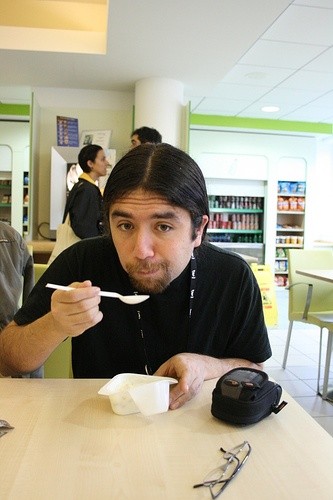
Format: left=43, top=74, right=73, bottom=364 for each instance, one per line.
left=98, top=373, right=179, bottom=415
left=277, top=236, right=303, bottom=244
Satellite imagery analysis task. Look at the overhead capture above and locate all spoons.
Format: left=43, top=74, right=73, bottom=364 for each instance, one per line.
left=46, top=283, right=149, bottom=304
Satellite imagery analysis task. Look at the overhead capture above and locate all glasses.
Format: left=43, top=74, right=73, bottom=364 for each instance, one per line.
left=193, top=441, right=252, bottom=499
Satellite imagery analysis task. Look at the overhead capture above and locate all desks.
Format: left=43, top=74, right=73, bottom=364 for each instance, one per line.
left=296, top=269, right=332, bottom=403
left=0, top=378, right=332, bottom=499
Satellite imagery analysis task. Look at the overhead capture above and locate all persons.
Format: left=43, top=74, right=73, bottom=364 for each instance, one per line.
left=0, top=143, right=273, bottom=410
left=62, top=145, right=113, bottom=242
left=130, top=127, right=162, bottom=147
left=0, top=222, right=44, bottom=378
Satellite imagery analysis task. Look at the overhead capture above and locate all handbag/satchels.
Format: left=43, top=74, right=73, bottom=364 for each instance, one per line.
left=47, top=212, right=82, bottom=264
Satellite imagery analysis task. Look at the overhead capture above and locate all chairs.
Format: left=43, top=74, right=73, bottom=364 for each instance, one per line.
left=283, top=249, right=333, bottom=394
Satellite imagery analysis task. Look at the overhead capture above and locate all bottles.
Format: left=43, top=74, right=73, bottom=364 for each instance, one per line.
left=207, top=196, right=263, bottom=209
left=206, top=213, right=260, bottom=230
left=206, top=233, right=263, bottom=242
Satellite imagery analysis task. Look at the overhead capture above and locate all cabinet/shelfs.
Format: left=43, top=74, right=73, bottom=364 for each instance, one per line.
left=196, top=154, right=306, bottom=289
